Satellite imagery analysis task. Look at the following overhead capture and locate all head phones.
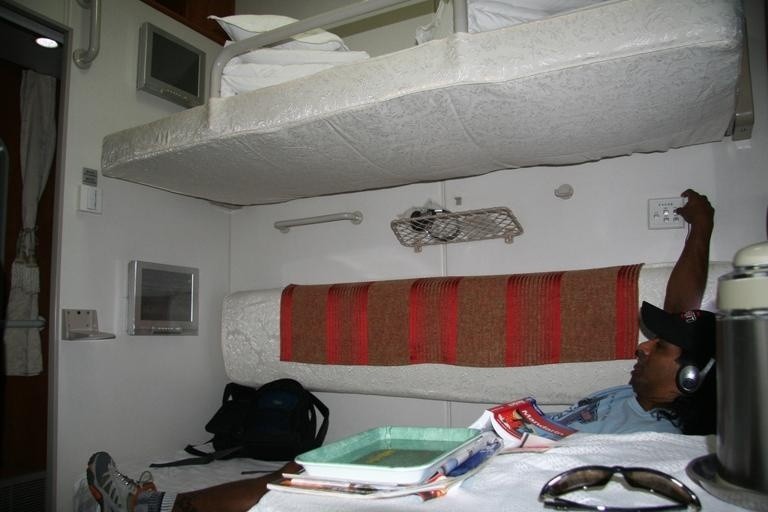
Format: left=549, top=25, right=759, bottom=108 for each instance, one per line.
left=675, top=355, right=717, bottom=395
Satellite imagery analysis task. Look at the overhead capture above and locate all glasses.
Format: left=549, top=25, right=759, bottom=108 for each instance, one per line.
left=538, top=465, right=702, bottom=512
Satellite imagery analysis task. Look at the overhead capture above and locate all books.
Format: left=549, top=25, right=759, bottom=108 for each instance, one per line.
left=468, top=396, right=578, bottom=445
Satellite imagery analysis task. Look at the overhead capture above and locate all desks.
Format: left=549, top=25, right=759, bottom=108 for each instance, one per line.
left=248, top=430, right=748, bottom=512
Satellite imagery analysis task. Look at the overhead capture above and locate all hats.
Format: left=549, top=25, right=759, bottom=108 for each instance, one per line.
left=642, top=301, right=716, bottom=358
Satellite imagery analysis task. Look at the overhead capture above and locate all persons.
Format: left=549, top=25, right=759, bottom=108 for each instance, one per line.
left=87, top=189, right=715, bottom=511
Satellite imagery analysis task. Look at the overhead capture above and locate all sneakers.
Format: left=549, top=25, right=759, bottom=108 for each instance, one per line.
left=87, top=452, right=156, bottom=511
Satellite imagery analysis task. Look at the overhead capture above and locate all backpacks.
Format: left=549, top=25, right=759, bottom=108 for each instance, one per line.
left=206, top=379, right=329, bottom=460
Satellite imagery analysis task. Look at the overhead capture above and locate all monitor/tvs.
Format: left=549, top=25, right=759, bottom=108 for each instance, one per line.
left=136, top=21, right=207, bottom=108
left=126, top=259, right=200, bottom=336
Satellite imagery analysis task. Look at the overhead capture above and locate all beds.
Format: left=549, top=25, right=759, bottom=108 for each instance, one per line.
left=73, top=255, right=732, bottom=511
left=100, top=0, right=756, bottom=207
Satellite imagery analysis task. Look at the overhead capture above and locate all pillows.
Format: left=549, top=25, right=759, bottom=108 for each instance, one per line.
left=414, top=0, right=600, bottom=44
left=205, top=14, right=350, bottom=51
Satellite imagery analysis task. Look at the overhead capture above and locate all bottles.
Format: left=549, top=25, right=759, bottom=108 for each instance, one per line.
left=714, top=242, right=768, bottom=495
